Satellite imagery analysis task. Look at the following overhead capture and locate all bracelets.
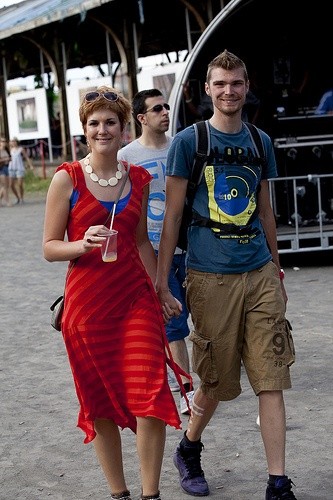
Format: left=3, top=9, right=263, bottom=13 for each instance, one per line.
left=279, top=268, right=284, bottom=280
left=185, top=98, right=193, bottom=104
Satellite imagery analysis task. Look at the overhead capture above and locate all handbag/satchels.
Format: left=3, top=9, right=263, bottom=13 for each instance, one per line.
left=50, top=295, right=64, bottom=331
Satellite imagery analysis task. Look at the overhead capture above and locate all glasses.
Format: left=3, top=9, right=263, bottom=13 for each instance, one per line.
left=82, top=91, right=122, bottom=102
left=143, top=103, right=170, bottom=114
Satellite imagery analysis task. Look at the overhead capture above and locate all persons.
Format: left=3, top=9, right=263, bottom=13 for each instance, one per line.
left=115, top=89, right=195, bottom=414
left=0, top=137, right=33, bottom=207
left=153, top=49, right=333, bottom=500
left=43, top=87, right=183, bottom=500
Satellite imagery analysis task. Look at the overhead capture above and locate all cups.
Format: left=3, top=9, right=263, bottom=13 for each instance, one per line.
left=96, top=230, right=118, bottom=262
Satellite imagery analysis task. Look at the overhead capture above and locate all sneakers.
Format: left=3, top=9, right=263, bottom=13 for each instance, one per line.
left=173, top=445, right=210, bottom=497
left=180, top=391, right=195, bottom=414
left=164, top=364, right=182, bottom=392
left=266, top=477, right=298, bottom=500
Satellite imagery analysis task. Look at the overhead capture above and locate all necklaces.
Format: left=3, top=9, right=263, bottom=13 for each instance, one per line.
left=84, top=153, right=122, bottom=187
